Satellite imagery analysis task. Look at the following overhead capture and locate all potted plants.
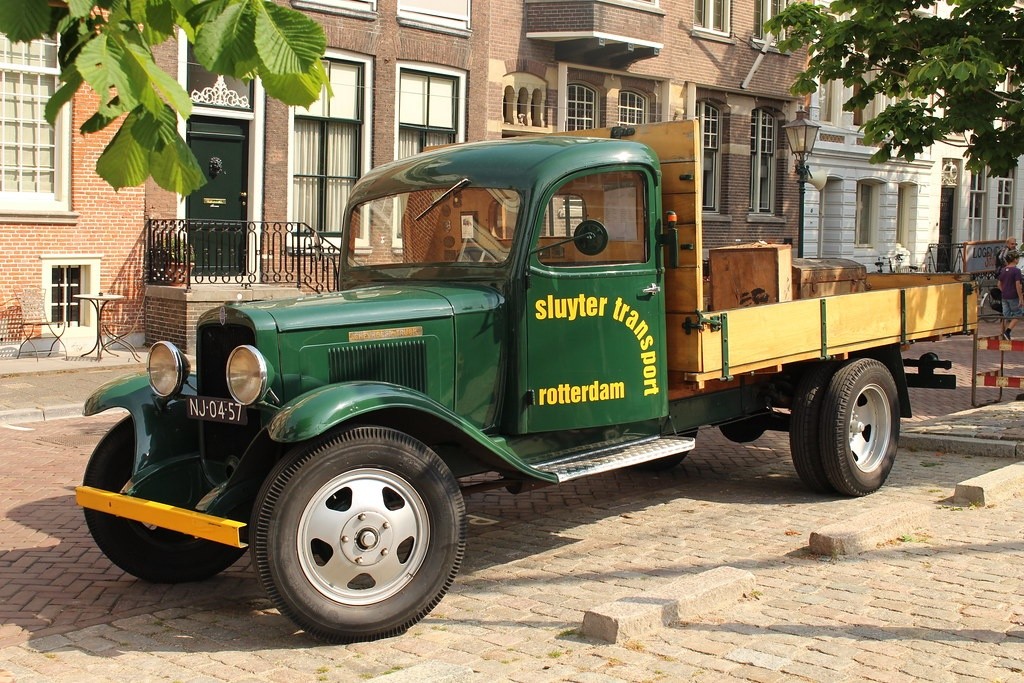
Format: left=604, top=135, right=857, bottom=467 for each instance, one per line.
left=145, top=236, right=195, bottom=286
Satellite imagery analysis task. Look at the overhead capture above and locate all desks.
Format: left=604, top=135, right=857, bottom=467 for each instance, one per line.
left=74, top=294, right=125, bottom=358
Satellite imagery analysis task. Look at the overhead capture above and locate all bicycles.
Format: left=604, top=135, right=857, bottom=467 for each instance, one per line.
left=875, top=254, right=918, bottom=273
left=980, top=288, right=1002, bottom=323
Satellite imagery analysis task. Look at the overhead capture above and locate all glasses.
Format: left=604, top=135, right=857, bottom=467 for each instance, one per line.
left=1008, top=242, right=1019, bottom=246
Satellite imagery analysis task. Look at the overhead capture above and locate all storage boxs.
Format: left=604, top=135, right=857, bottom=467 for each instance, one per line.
left=703, top=243, right=867, bottom=312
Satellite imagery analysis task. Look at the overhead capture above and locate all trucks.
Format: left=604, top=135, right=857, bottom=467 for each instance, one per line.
left=77, top=117, right=978, bottom=641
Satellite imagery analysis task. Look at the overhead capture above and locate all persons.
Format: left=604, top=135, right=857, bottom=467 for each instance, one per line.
left=994, top=237, right=1024, bottom=340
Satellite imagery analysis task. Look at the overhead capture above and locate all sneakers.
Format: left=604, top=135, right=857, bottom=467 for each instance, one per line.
left=1003, top=331, right=1011, bottom=341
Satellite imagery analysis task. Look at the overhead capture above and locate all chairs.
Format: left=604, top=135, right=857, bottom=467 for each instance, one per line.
left=13, top=285, right=67, bottom=361
left=100, top=287, right=148, bottom=363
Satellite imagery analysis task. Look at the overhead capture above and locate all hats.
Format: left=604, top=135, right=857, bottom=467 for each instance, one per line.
left=1007, top=250, right=1021, bottom=258
